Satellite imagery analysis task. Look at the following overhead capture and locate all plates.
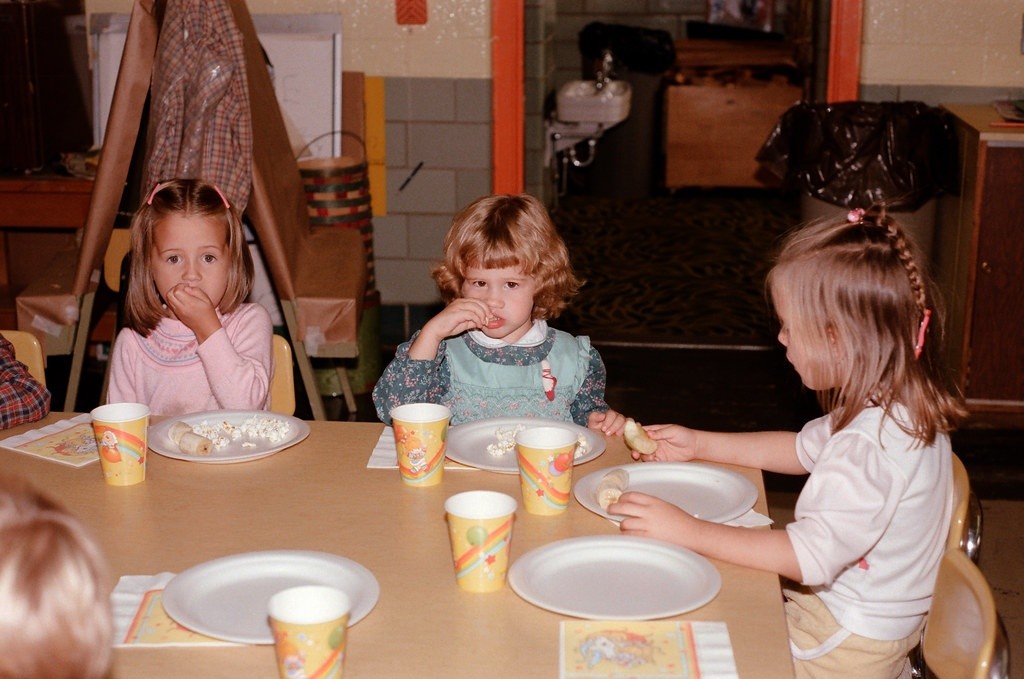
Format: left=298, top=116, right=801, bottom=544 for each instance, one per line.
left=148, top=409, right=310, bottom=464
left=573, top=461, right=760, bottom=524
left=164, top=548, right=382, bottom=645
left=506, top=535, right=722, bottom=616
left=446, top=416, right=607, bottom=475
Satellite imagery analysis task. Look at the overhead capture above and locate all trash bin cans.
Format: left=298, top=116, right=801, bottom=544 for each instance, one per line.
left=799, top=103, right=935, bottom=264
left=578, top=22, right=669, bottom=200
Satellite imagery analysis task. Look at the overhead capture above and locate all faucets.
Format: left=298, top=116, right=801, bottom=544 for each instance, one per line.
left=593, top=49, right=613, bottom=93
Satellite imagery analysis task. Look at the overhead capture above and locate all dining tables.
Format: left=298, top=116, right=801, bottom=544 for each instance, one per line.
left=2, top=411, right=796, bottom=679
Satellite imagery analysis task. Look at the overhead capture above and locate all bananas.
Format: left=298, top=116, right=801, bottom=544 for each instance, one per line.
left=596, top=468, right=628, bottom=508
left=168, top=421, right=213, bottom=457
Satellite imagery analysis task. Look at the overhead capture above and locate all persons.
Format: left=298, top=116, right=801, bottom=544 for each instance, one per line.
left=607, top=208, right=955, bottom=679
left=106, top=178, right=275, bottom=420
left=371, top=194, right=642, bottom=436
left=0, top=472, right=113, bottom=679
left=0, top=333, right=50, bottom=434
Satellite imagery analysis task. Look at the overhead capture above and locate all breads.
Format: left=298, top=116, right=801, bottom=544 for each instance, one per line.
left=623, top=420, right=657, bottom=454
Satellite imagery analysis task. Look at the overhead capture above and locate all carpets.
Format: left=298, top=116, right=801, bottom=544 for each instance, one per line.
left=542, top=193, right=800, bottom=353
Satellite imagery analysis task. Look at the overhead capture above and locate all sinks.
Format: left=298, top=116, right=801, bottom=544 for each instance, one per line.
left=555, top=80, right=631, bottom=136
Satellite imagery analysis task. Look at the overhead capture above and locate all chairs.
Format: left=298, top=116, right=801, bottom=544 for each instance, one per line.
left=912, top=552, right=1011, bottom=679
left=945, top=451, right=985, bottom=560
left=1, top=327, right=296, bottom=416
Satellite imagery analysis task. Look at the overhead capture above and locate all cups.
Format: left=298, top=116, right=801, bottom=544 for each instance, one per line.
left=90, top=401, right=147, bottom=486
left=269, top=584, right=352, bottom=679
left=443, top=489, right=517, bottom=596
left=389, top=401, right=453, bottom=489
left=514, top=427, right=578, bottom=516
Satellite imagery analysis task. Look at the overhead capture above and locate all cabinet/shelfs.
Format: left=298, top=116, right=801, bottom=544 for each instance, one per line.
left=665, top=83, right=802, bottom=191
left=928, top=100, right=1024, bottom=427
